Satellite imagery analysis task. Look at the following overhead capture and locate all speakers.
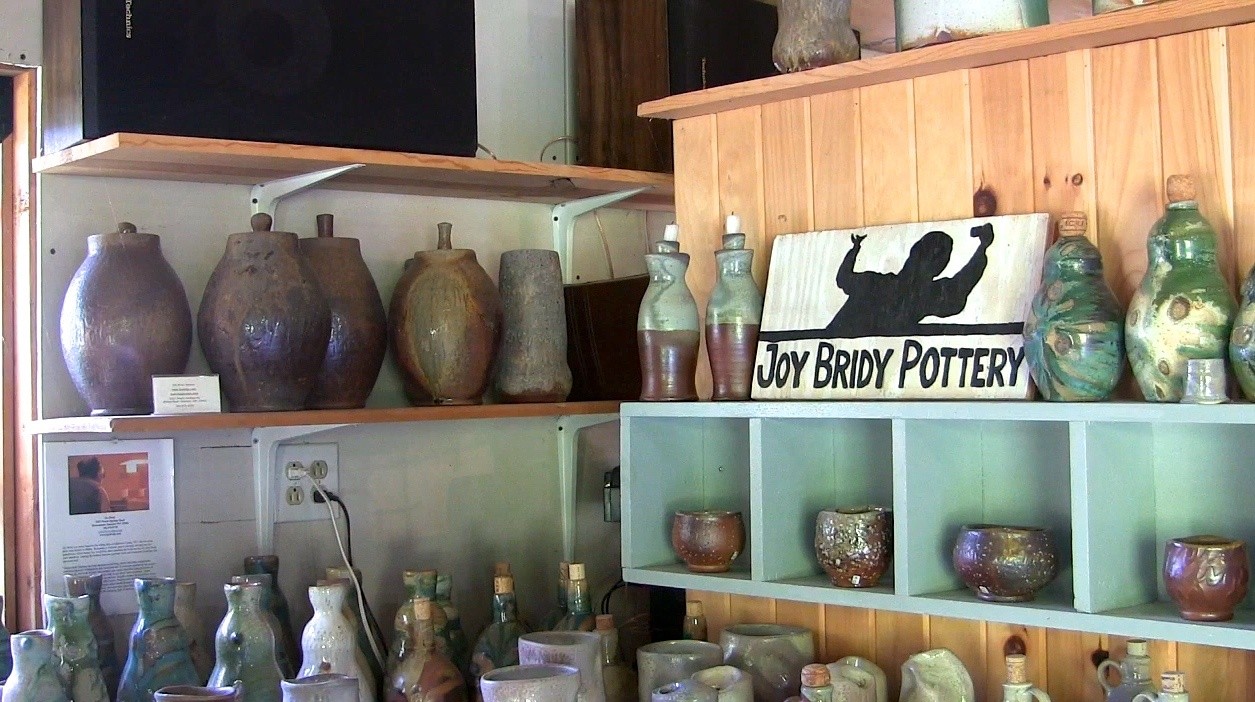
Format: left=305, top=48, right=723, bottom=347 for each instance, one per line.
left=41, top=0, right=478, bottom=158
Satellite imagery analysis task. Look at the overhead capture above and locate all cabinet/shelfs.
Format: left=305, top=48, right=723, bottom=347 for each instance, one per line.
left=37, top=1, right=1255, bottom=654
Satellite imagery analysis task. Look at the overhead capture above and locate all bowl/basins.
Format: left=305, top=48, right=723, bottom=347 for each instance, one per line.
left=811, top=506, right=890, bottom=586
left=669, top=509, right=746, bottom=575
left=951, top=522, right=1060, bottom=602
left=1163, top=534, right=1250, bottom=623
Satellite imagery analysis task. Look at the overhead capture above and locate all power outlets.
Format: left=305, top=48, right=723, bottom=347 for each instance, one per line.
left=273, top=444, right=340, bottom=522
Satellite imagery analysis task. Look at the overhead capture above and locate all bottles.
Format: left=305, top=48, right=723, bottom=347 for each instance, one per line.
left=1, top=173, right=1255, bottom=702
